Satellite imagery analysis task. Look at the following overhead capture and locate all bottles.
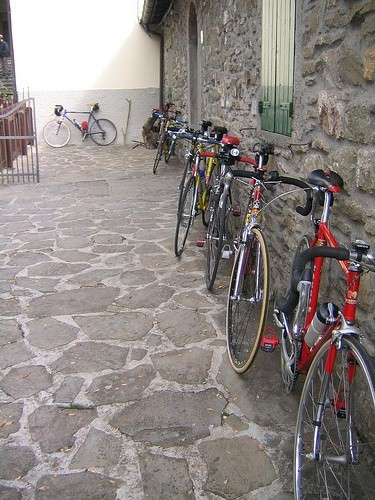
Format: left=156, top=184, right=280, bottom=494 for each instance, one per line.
left=303, top=302, right=340, bottom=348
left=200, top=169, right=206, bottom=192
left=82, top=121, right=88, bottom=130
left=73, top=119, right=81, bottom=127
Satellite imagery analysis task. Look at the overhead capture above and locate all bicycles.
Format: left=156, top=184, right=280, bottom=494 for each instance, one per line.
left=150, top=102, right=229, bottom=258
left=224, top=141, right=314, bottom=374
left=195, top=135, right=258, bottom=290
left=257, top=168, right=375, bottom=500
left=42, top=102, right=117, bottom=148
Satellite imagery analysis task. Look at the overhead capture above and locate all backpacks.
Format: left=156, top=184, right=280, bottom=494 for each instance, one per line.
left=142, top=112, right=160, bottom=149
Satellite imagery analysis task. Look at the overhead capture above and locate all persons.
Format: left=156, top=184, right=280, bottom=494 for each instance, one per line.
left=0, top=33, right=9, bottom=79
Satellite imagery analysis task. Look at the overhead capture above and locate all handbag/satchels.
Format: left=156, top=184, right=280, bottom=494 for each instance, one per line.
left=55, top=105, right=63, bottom=116
